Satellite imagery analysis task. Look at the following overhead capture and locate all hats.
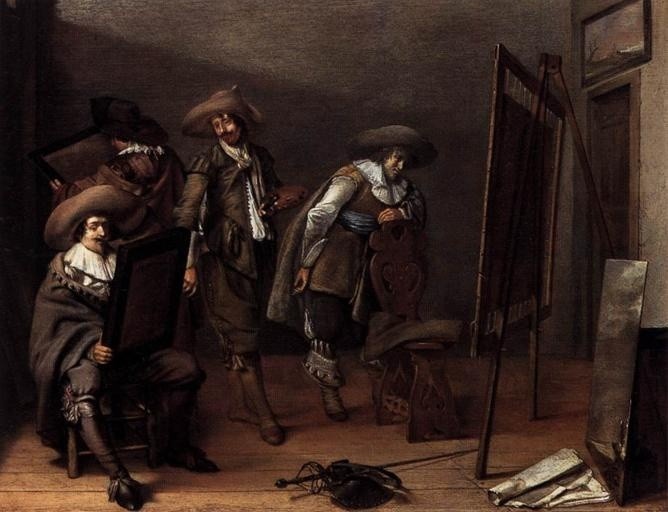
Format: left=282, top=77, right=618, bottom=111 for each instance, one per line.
left=344, top=125, right=438, bottom=169
left=181, top=90, right=260, bottom=139
left=43, top=184, right=148, bottom=251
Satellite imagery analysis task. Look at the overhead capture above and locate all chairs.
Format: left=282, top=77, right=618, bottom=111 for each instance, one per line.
left=367, top=220, right=463, bottom=442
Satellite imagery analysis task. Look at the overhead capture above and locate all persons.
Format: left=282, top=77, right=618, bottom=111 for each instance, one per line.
left=44, top=112, right=187, bottom=246
left=172, top=111, right=286, bottom=446
left=26, top=212, right=221, bottom=511
left=291, top=145, right=429, bottom=425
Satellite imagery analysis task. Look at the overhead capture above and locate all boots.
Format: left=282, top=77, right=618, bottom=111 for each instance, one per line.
left=240, top=365, right=285, bottom=445
left=226, top=368, right=260, bottom=425
left=321, top=385, right=347, bottom=421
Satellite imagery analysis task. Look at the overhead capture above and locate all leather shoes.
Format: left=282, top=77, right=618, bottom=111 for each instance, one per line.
left=108, top=466, right=143, bottom=511
left=163, top=441, right=218, bottom=473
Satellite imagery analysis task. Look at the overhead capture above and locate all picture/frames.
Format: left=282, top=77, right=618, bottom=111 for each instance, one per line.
left=579, top=0, right=653, bottom=90
left=99, top=226, right=191, bottom=365
